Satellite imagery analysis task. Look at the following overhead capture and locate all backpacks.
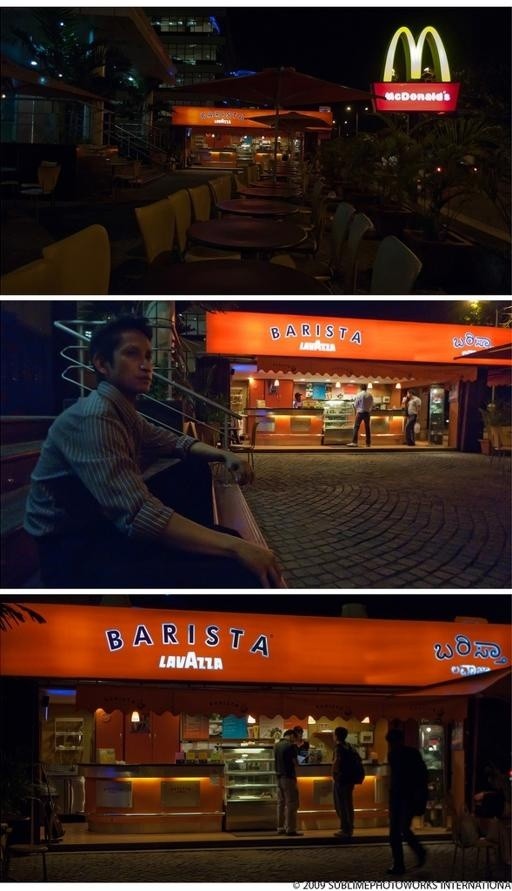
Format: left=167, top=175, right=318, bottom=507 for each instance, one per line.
left=336, top=743, right=365, bottom=785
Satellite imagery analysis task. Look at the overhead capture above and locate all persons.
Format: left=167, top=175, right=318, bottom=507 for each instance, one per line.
left=381, top=727, right=428, bottom=873
left=401, top=388, right=422, bottom=445
left=293, top=725, right=311, bottom=763
left=23, top=311, right=281, bottom=589
left=273, top=727, right=305, bottom=835
left=346, top=383, right=374, bottom=446
left=333, top=725, right=360, bottom=836
left=293, top=391, right=304, bottom=408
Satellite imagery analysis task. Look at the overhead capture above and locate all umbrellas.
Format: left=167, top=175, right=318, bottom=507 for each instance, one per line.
left=244, top=107, right=328, bottom=135
left=170, top=60, right=387, bottom=177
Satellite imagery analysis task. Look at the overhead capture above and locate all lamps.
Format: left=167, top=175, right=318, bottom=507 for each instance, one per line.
left=272, top=378, right=404, bottom=391
left=123, top=706, right=374, bottom=727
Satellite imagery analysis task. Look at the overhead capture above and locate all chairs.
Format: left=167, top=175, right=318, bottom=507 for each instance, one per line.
left=1, top=139, right=510, bottom=295
left=444, top=803, right=512, bottom=881
left=184, top=421, right=260, bottom=467
left=486, top=426, right=512, bottom=473
left=9, top=802, right=55, bottom=882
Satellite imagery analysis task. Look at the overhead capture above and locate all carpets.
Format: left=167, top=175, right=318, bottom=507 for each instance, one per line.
left=233, top=830, right=289, bottom=838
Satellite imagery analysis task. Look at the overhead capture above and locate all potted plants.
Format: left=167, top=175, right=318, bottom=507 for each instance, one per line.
left=479, top=439, right=491, bottom=455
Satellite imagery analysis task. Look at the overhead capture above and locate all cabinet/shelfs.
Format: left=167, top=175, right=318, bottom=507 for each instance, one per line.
left=322, top=398, right=359, bottom=447
left=215, top=742, right=277, bottom=833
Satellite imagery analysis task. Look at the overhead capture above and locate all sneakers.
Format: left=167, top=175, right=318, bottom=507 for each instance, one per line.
left=415, top=845, right=428, bottom=866
left=277, top=827, right=304, bottom=837
left=333, top=829, right=353, bottom=839
left=385, top=867, right=406, bottom=874
